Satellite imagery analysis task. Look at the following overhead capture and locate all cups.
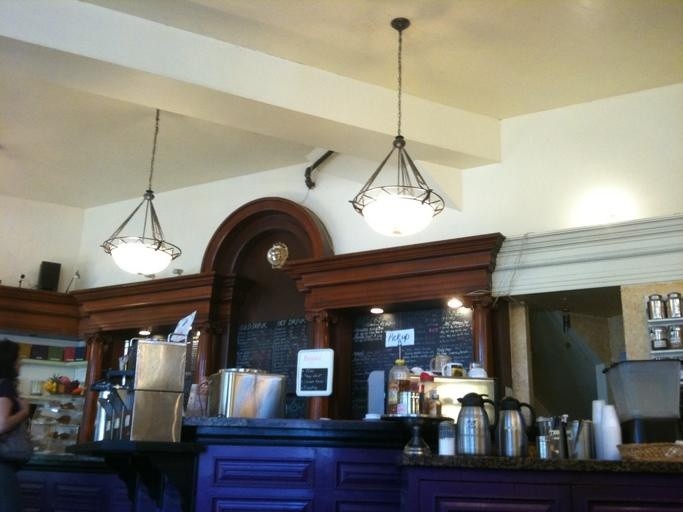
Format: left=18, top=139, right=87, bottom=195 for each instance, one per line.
left=591, top=396, right=623, bottom=461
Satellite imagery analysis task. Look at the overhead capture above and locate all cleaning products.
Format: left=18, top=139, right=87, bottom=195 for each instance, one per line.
left=389, top=359, right=411, bottom=415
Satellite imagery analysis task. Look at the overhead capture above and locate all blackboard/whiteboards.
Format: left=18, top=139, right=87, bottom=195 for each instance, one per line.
left=236, top=317, right=307, bottom=417
left=352, top=308, right=473, bottom=419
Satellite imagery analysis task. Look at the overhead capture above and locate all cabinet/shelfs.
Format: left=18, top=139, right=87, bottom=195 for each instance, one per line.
left=643, top=314, right=683, bottom=359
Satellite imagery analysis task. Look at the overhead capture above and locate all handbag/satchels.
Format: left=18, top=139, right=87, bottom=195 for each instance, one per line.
left=0, top=432, right=32, bottom=463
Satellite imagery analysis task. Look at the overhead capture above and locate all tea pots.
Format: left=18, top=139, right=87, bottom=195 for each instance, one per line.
left=429, top=353, right=454, bottom=375
left=441, top=362, right=466, bottom=378
left=454, top=391, right=498, bottom=457
left=495, top=394, right=536, bottom=457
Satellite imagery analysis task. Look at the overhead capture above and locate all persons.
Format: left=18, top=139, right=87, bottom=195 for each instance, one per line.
left=0, top=339, right=31, bottom=434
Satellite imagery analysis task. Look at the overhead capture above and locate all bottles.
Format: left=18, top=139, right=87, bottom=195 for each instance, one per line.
left=648, top=325, right=669, bottom=349
left=668, top=323, right=683, bottom=349
left=647, top=294, right=667, bottom=319
left=385, top=358, right=442, bottom=415
left=438, top=420, right=456, bottom=455
left=664, top=291, right=683, bottom=318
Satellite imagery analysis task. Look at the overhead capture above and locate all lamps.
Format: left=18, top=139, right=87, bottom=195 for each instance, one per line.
left=347, top=13, right=445, bottom=240
left=96, top=102, right=182, bottom=277
